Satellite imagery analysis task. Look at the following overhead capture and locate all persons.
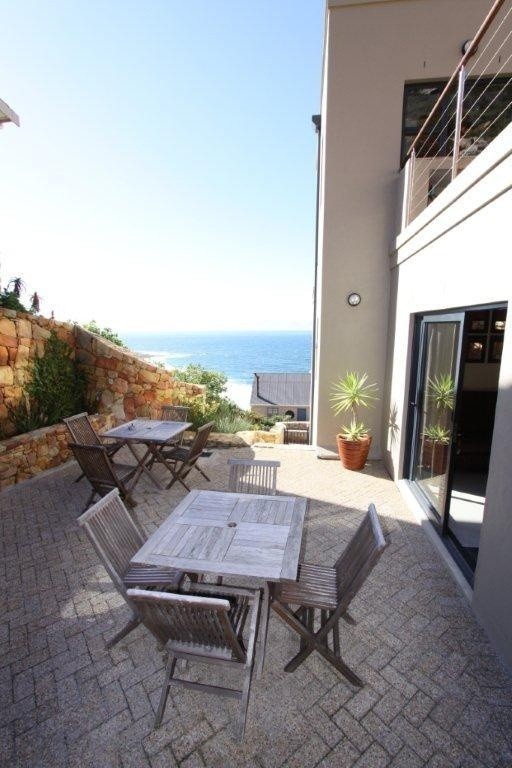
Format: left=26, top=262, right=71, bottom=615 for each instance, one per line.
left=407, top=110, right=441, bottom=158
left=460, top=104, right=492, bottom=146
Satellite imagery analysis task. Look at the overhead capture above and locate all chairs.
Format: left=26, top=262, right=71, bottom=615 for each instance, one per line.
left=227, top=458, right=281, bottom=496
left=149, top=406, right=188, bottom=470
left=271, top=503, right=388, bottom=686
left=67, top=441, right=142, bottom=516
left=75, top=487, right=187, bottom=650
left=63, top=412, right=119, bottom=483
left=162, top=420, right=216, bottom=491
left=126, top=580, right=264, bottom=741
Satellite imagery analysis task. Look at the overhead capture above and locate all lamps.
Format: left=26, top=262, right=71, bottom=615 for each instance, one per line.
left=348, top=293, right=361, bottom=306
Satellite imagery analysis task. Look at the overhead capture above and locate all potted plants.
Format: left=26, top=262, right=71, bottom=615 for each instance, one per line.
left=327, top=368, right=379, bottom=470
left=420, top=372, right=454, bottom=479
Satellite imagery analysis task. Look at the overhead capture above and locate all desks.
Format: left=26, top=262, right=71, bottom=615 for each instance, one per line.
left=99, top=417, right=193, bottom=491
left=129, top=489, right=307, bottom=680
